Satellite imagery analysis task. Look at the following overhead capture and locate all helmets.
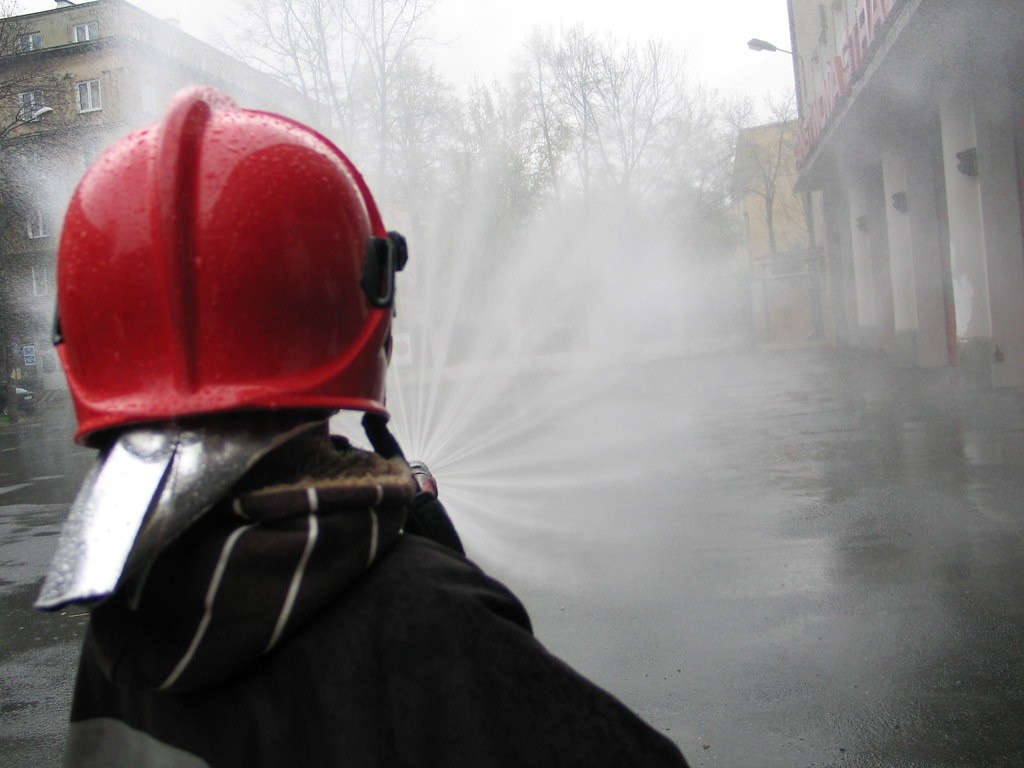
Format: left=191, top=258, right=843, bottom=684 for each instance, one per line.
left=51, top=84, right=411, bottom=443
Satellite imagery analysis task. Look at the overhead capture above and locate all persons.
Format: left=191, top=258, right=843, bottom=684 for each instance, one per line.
left=33, top=88, right=691, bottom=768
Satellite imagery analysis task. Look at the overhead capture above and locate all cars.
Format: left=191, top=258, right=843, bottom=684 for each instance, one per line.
left=0, top=381, right=38, bottom=418
